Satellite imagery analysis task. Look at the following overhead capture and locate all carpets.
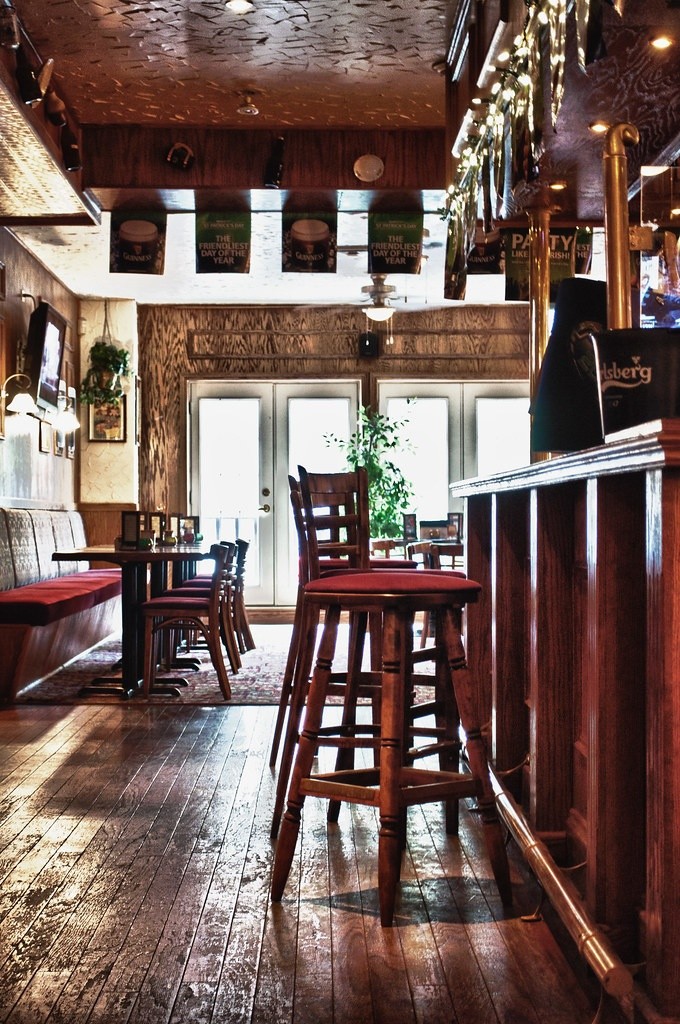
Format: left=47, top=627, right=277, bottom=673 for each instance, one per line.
left=15, top=622, right=438, bottom=705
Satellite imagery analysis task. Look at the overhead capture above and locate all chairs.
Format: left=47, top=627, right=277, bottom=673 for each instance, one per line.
left=161, top=540, right=242, bottom=674
left=138, top=544, right=231, bottom=701
left=193, top=538, right=256, bottom=652
left=407, top=540, right=433, bottom=568
left=419, top=544, right=463, bottom=649
left=183, top=543, right=239, bottom=656
left=366, top=536, right=395, bottom=559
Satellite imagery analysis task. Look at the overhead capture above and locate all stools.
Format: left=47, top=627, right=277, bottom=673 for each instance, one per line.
left=269, top=470, right=416, bottom=765
left=272, top=572, right=514, bottom=927
left=268, top=466, right=460, bottom=841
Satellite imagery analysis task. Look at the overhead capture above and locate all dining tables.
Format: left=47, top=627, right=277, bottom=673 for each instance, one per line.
left=52, top=536, right=226, bottom=702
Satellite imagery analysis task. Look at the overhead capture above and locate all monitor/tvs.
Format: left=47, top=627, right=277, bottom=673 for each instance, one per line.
left=25, top=304, right=69, bottom=415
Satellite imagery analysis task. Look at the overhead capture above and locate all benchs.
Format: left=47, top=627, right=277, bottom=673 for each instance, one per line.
left=0, top=508, right=121, bottom=628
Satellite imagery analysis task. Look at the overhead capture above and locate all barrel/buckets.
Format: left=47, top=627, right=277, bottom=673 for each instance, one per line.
left=590, top=327, right=680, bottom=439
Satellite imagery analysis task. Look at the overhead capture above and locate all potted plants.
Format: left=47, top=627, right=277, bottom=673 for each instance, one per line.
left=79, top=341, right=129, bottom=404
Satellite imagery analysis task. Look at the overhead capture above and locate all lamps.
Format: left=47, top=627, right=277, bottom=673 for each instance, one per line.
left=0, top=4, right=20, bottom=50
left=549, top=180, right=566, bottom=190
left=45, top=86, right=66, bottom=128
left=588, top=120, right=610, bottom=133
left=61, top=125, right=83, bottom=172
left=353, top=131, right=383, bottom=181
left=236, top=96, right=259, bottom=116
left=224, top=0, right=255, bottom=12
left=263, top=136, right=286, bottom=189
left=2, top=373, right=40, bottom=415
left=360, top=273, right=406, bottom=343
left=14, top=44, right=42, bottom=105
left=162, top=142, right=196, bottom=170
left=648, top=34, right=676, bottom=50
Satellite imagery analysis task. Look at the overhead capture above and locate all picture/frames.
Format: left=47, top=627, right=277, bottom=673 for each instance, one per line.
left=52, top=430, right=66, bottom=456
left=86, top=394, right=127, bottom=443
left=66, top=428, right=76, bottom=460
left=39, top=421, right=53, bottom=454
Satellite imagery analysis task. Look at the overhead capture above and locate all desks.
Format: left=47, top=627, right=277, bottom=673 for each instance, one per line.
left=448, top=417, right=680, bottom=1024
